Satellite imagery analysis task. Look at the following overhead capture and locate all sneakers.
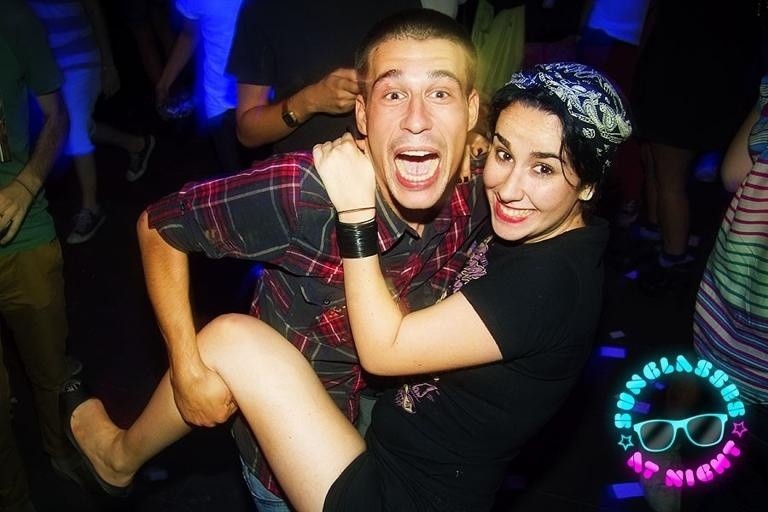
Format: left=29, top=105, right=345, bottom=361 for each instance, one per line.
left=125, top=134, right=155, bottom=182
left=66, top=206, right=108, bottom=245
left=615, top=200, right=642, bottom=228
left=630, top=250, right=701, bottom=297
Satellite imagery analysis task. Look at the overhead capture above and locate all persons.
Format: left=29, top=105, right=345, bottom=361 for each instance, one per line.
left=0, top=0, right=766, bottom=511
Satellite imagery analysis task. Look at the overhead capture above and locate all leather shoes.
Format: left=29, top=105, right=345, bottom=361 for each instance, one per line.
left=58, top=379, right=135, bottom=498
